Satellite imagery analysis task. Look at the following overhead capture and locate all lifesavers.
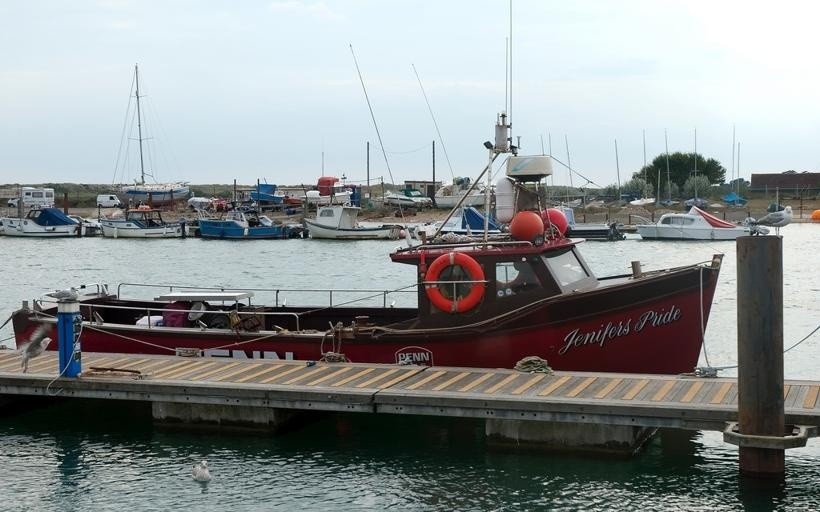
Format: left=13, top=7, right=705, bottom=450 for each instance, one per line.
left=424, top=253, right=486, bottom=313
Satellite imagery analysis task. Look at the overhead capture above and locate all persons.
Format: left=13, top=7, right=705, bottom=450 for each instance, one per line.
left=129, top=198, right=144, bottom=209
left=209, top=200, right=224, bottom=213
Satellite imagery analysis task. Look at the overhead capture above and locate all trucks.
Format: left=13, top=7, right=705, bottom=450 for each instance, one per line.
left=7, top=187, right=55, bottom=208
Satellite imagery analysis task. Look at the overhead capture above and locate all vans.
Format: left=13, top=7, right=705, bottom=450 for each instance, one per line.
left=97, top=195, right=121, bottom=208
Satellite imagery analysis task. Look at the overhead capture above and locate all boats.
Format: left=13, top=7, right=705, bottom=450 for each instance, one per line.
left=304, top=186, right=402, bottom=242
left=434, top=176, right=496, bottom=209
left=636, top=205, right=755, bottom=242
left=1, top=206, right=102, bottom=239
left=98, top=204, right=191, bottom=240
left=12, top=112, right=725, bottom=375
left=414, top=203, right=509, bottom=234
left=198, top=178, right=308, bottom=241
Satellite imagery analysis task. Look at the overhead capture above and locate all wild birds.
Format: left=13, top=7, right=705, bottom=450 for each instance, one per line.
left=756, top=205, right=794, bottom=240
left=42, top=286, right=79, bottom=302
left=191, top=460, right=212, bottom=483
left=15, top=322, right=54, bottom=374
left=744, top=211, right=770, bottom=236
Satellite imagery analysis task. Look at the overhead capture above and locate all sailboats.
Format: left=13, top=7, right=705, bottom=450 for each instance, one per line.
left=112, top=63, right=192, bottom=205
